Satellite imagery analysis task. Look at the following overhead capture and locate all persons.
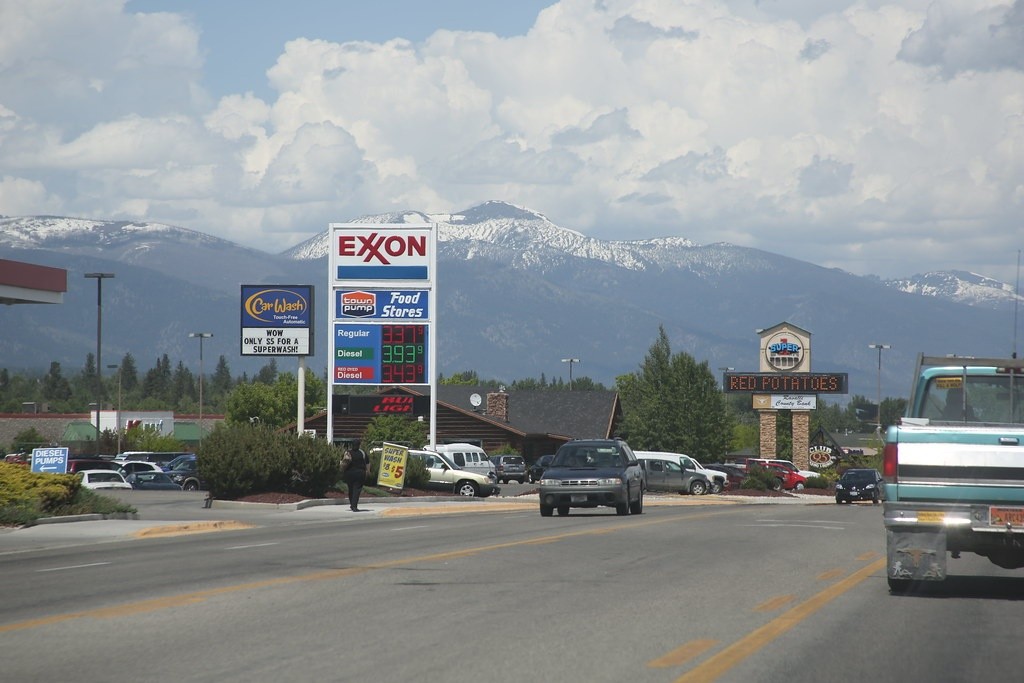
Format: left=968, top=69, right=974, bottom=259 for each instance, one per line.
left=336, top=439, right=369, bottom=512
left=943, top=387, right=969, bottom=404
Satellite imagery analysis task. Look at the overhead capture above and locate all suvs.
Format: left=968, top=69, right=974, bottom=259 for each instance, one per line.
left=539, top=437, right=644, bottom=517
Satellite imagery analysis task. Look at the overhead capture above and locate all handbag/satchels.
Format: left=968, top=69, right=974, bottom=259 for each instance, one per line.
left=340, top=451, right=352, bottom=470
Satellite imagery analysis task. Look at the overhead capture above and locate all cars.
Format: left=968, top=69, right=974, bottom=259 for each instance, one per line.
left=370, top=443, right=554, bottom=497
left=836, top=469, right=882, bottom=504
left=5, top=443, right=207, bottom=491
left=633, top=451, right=822, bottom=495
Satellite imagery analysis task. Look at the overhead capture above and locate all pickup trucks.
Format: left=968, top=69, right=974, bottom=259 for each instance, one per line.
left=883, top=364, right=1024, bottom=594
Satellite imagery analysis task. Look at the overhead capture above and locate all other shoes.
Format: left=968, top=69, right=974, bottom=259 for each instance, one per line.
left=350, top=506, right=358, bottom=512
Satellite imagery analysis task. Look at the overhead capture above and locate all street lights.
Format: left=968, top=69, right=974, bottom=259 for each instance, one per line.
left=721, top=367, right=735, bottom=372
left=869, top=344, right=891, bottom=454
left=107, top=365, right=132, bottom=454
left=189, top=333, right=213, bottom=449
left=562, top=359, right=580, bottom=391
